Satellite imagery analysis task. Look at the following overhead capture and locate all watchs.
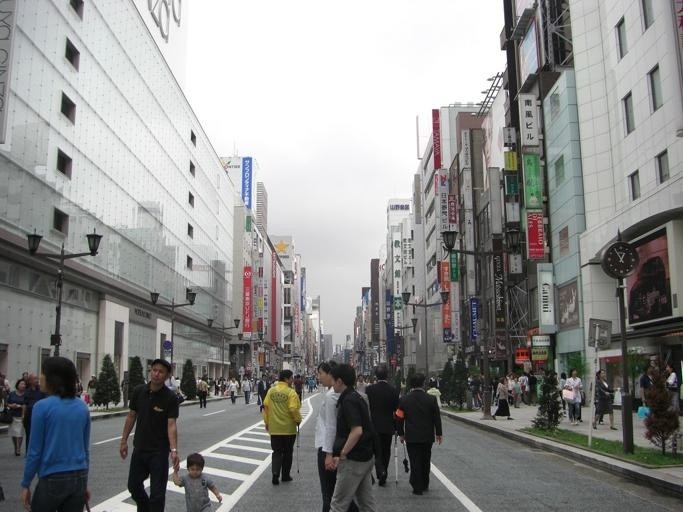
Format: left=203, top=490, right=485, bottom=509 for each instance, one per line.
left=340, top=449, right=346, bottom=456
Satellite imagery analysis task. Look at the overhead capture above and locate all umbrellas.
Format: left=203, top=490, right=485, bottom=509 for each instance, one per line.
left=401, top=441, right=409, bottom=475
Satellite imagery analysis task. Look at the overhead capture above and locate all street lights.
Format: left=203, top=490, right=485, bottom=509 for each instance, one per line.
left=400, top=291, right=449, bottom=384
left=148, top=288, right=197, bottom=374
left=26, top=228, right=102, bottom=355
left=206, top=318, right=239, bottom=381
left=383, top=318, right=417, bottom=382
left=238, top=333, right=263, bottom=373
left=441, top=229, right=519, bottom=420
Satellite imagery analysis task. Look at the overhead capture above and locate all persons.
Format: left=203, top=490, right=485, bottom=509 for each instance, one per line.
left=294, top=374, right=318, bottom=396
left=195, top=375, right=271, bottom=408
left=364, top=364, right=397, bottom=486
left=166, top=376, right=181, bottom=391
left=470, top=372, right=538, bottom=420
left=592, top=371, right=619, bottom=430
left=313, top=359, right=359, bottom=512
left=628, top=256, right=672, bottom=325
left=329, top=362, right=377, bottom=512
left=428, top=376, right=446, bottom=408
left=1, top=373, right=44, bottom=456
left=118, top=358, right=179, bottom=511
left=75, top=374, right=98, bottom=405
left=639, top=362, right=682, bottom=413
left=541, top=369, right=585, bottom=423
left=172, top=453, right=222, bottom=512
left=19, top=356, right=91, bottom=512
left=261, top=368, right=302, bottom=486
left=558, top=288, right=568, bottom=309
left=397, top=374, right=443, bottom=496
left=362, top=377, right=376, bottom=386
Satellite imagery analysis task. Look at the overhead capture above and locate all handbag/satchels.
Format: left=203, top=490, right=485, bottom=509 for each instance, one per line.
left=562, top=389, right=574, bottom=401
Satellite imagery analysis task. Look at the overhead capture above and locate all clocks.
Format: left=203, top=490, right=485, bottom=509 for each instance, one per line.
left=600, top=242, right=639, bottom=279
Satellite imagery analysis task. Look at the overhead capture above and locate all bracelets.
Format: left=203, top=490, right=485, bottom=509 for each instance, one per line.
left=120, top=437, right=127, bottom=441
left=169, top=448, right=178, bottom=453
